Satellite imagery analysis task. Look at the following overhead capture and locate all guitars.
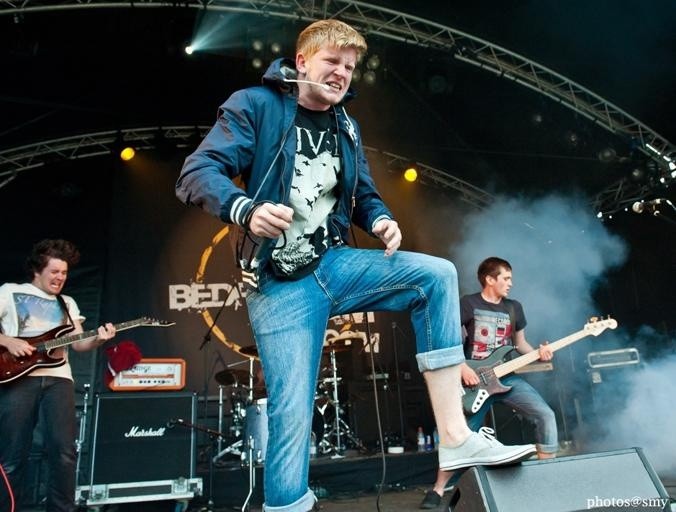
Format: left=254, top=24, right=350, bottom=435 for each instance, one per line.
left=0, top=314, right=177, bottom=385
left=462, top=315, right=618, bottom=416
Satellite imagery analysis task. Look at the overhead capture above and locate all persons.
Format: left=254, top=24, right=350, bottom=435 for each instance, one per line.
left=174, top=19, right=538, bottom=512
left=0, top=240, right=116, bottom=512
left=421, top=256, right=558, bottom=508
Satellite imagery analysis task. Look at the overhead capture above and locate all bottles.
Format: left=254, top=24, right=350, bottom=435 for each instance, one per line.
left=416, top=427, right=439, bottom=452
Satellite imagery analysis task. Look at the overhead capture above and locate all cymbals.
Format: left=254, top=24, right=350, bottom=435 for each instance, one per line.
left=214, top=369, right=259, bottom=385
left=239, top=345, right=258, bottom=357
left=321, top=343, right=354, bottom=354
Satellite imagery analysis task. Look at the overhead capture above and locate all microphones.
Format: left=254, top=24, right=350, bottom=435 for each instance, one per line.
left=284, top=79, right=330, bottom=91
left=168, top=415, right=177, bottom=429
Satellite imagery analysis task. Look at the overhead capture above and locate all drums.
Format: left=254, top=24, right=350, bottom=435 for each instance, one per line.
left=309, top=405, right=327, bottom=446
left=313, top=376, right=344, bottom=407
left=240, top=398, right=317, bottom=469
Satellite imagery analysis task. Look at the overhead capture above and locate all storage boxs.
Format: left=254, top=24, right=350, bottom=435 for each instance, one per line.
left=91, top=392, right=197, bottom=502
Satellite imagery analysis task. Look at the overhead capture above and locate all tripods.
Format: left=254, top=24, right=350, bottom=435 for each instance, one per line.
left=211, top=359, right=254, bottom=460
left=321, top=350, right=366, bottom=455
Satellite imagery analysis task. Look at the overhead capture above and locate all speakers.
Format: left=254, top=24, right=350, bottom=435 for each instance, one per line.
left=443, top=446, right=672, bottom=512
left=88, top=391, right=197, bottom=483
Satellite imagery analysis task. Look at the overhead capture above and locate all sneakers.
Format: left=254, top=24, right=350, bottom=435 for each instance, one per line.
left=420, top=489, right=442, bottom=508
left=438, top=426, right=538, bottom=473
